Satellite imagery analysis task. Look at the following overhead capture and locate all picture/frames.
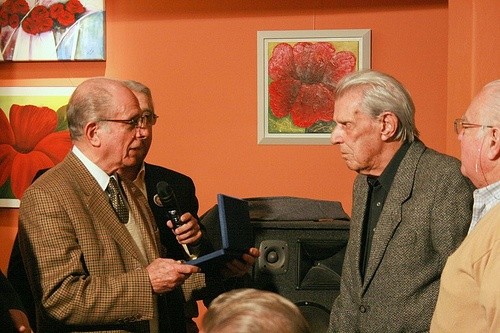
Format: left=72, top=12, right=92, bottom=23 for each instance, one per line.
left=1, top=77, right=103, bottom=208
left=257, top=29, right=371, bottom=146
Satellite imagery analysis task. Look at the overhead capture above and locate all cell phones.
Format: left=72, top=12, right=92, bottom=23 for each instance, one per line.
left=157, top=182, right=184, bottom=233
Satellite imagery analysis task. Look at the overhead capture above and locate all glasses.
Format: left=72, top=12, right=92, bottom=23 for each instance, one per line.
left=97, top=117, right=147, bottom=127
left=144, top=113, right=159, bottom=125
left=454, top=118, right=494, bottom=133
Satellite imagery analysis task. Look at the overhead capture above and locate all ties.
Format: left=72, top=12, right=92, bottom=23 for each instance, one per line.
left=105, top=176, right=129, bottom=223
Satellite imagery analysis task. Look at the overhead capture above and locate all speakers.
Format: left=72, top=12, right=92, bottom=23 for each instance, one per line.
left=245, top=220, right=352, bottom=333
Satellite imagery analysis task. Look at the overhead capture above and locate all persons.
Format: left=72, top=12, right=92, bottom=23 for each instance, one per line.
left=431, top=77, right=500, bottom=333
left=0, top=79, right=260, bottom=333
left=327, top=71, right=480, bottom=333
left=202, top=288, right=311, bottom=333
